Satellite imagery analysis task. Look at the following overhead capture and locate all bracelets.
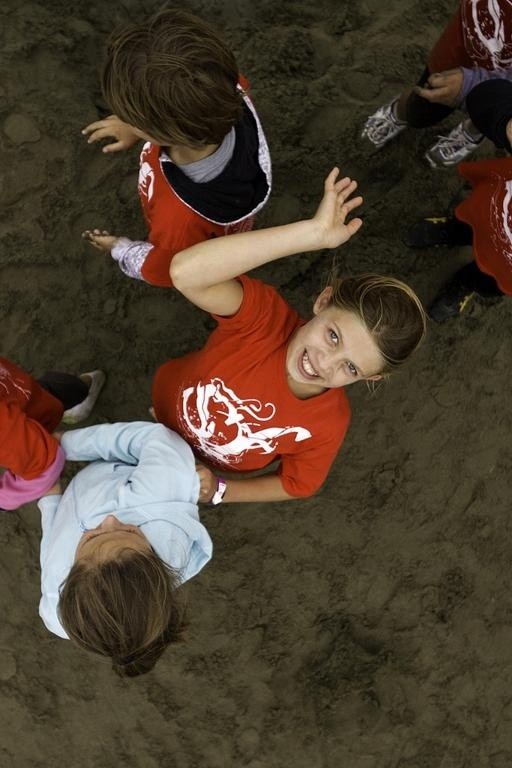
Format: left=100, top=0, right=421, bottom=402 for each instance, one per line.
left=210, top=476, right=226, bottom=508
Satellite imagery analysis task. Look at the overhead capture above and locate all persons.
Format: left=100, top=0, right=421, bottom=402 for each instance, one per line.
left=73, top=6, right=275, bottom=288
left=28, top=418, right=218, bottom=678
left=402, top=76, right=511, bottom=326
left=3, top=356, right=105, bottom=522
left=152, top=165, right=425, bottom=509
left=364, top=0, right=512, bottom=169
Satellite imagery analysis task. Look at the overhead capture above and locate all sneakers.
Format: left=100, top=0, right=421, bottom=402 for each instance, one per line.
left=422, top=119, right=487, bottom=171
left=360, top=93, right=408, bottom=152
left=403, top=216, right=454, bottom=249
left=61, top=369, right=106, bottom=425
left=431, top=280, right=479, bottom=323
